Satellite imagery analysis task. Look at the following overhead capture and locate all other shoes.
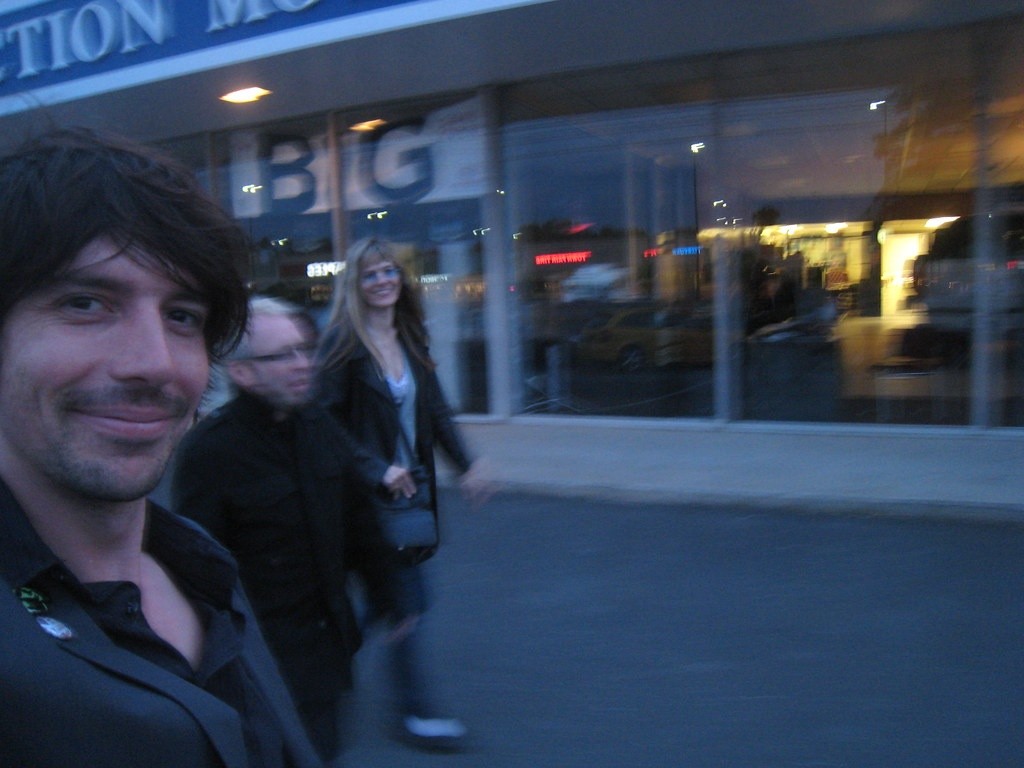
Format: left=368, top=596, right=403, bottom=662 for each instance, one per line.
left=389, top=705, right=465, bottom=751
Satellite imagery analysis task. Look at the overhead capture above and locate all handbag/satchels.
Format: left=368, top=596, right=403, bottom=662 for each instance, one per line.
left=372, top=464, right=438, bottom=553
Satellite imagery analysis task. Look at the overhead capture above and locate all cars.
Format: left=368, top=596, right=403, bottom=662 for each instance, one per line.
left=524, top=293, right=717, bottom=380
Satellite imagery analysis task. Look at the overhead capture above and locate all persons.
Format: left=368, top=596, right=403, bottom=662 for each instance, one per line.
left=744, top=263, right=832, bottom=361
left=172, top=236, right=473, bottom=756
left=0, top=125, right=321, bottom=768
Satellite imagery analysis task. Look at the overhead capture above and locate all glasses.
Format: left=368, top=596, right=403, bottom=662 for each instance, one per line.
left=241, top=342, right=312, bottom=364
left=357, top=268, right=401, bottom=282
